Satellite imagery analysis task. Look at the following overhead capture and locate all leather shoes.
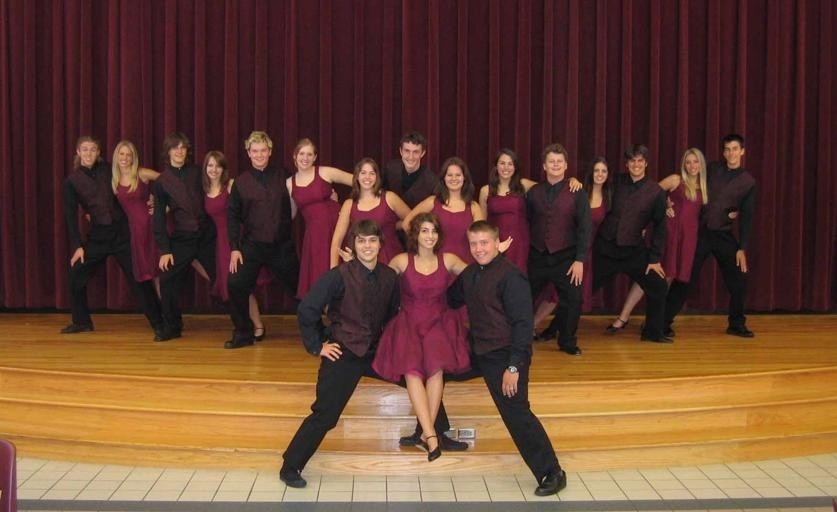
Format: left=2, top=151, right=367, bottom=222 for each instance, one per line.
left=542, top=318, right=760, bottom=356
left=401, top=429, right=471, bottom=462
left=150, top=323, right=271, bottom=348
left=56, top=322, right=98, bottom=335
left=276, top=462, right=311, bottom=487
left=534, top=469, right=567, bottom=496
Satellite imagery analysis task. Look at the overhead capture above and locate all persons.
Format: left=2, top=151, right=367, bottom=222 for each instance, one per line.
left=280, top=213, right=567, bottom=497
left=60, top=128, right=754, bottom=355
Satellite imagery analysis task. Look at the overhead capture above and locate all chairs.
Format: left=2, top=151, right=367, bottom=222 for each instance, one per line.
left=0, top=438, right=17, bottom=512
